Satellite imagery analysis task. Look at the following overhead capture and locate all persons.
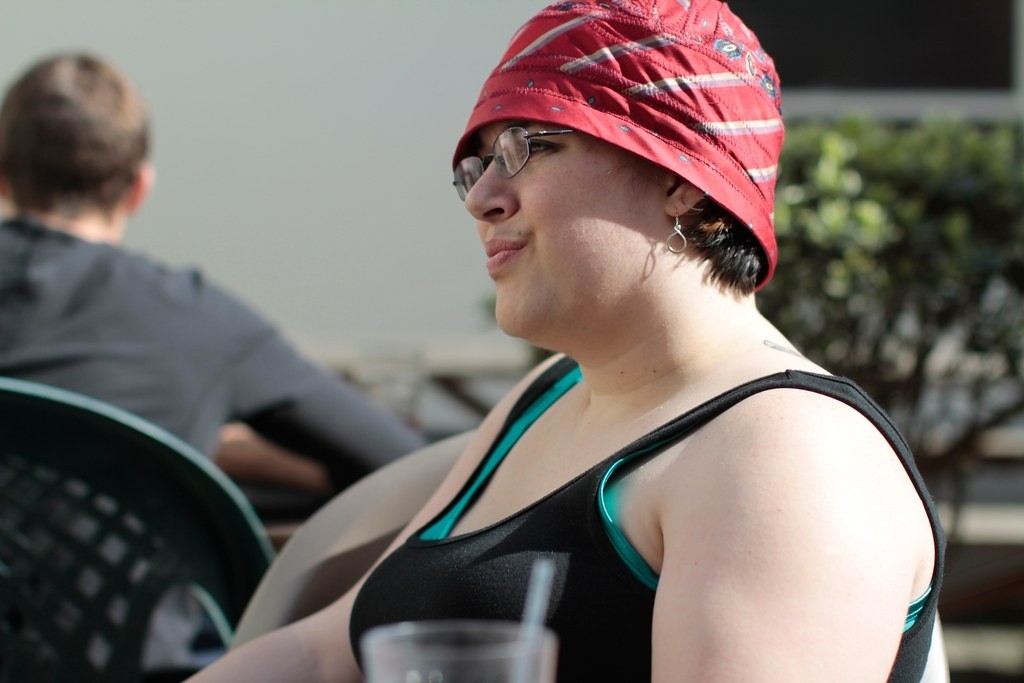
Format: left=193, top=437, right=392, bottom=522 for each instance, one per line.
left=0, top=52, right=430, bottom=502
left=183, top=1, right=946, bottom=683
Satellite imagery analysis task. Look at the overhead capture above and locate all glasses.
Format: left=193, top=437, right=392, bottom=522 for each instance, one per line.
left=453, top=127, right=576, bottom=193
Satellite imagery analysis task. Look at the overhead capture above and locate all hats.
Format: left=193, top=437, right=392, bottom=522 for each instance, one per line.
left=454, top=0, right=786, bottom=289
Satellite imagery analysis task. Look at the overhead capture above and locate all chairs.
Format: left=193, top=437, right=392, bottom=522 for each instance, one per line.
left=0, top=377, right=279, bottom=683
left=227, top=425, right=951, bottom=683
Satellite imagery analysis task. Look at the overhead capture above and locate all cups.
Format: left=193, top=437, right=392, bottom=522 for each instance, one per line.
left=358, top=616, right=558, bottom=683
